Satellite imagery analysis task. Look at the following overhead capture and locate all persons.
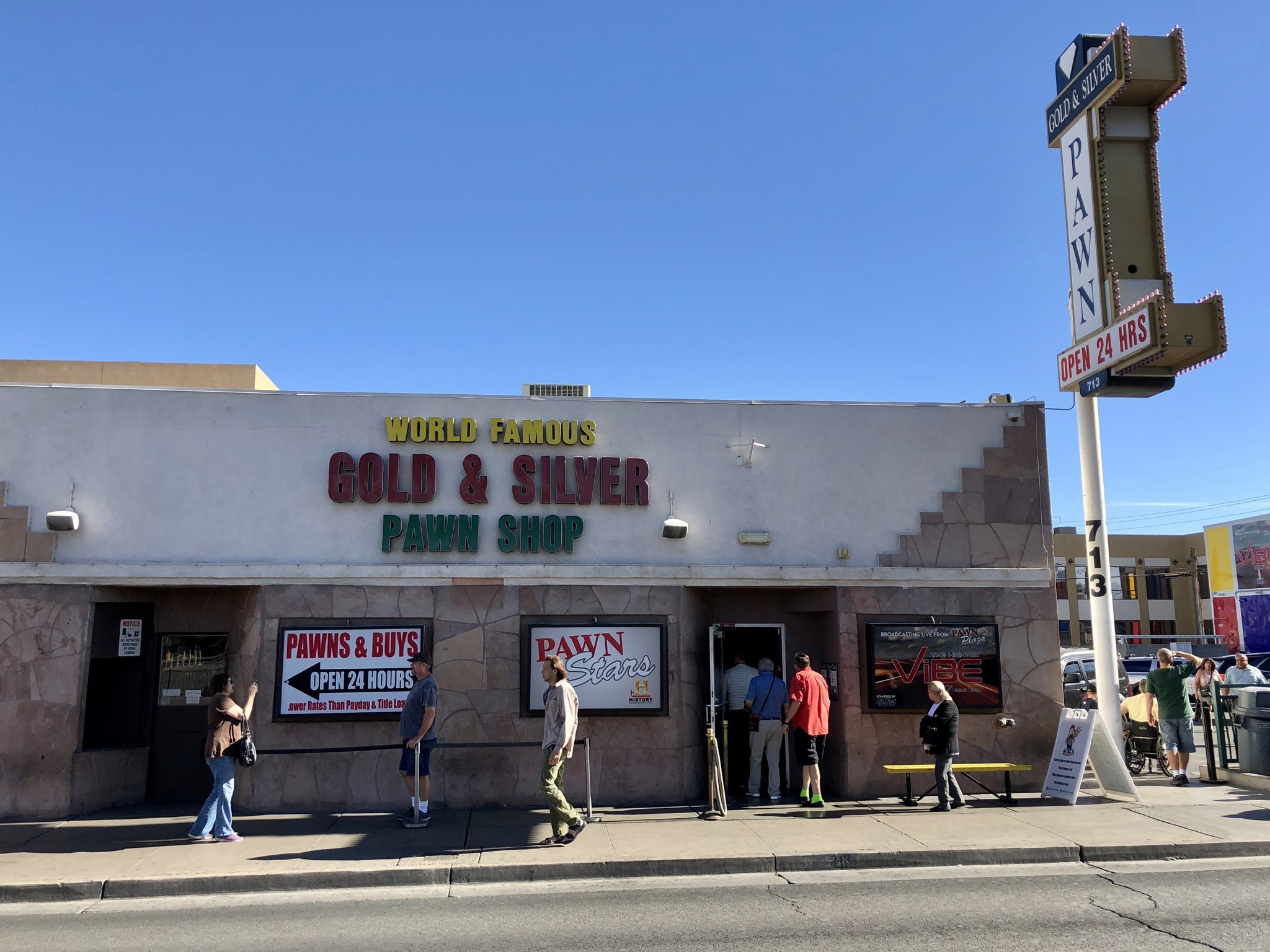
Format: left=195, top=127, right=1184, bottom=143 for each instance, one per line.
left=1144, top=648, right=1203, bottom=785
left=188, top=673, right=258, bottom=842
left=397, top=652, right=439, bottom=821
left=723, top=649, right=760, bottom=787
left=1121, top=679, right=1171, bottom=770
left=1117, top=652, right=1121, bottom=658
left=1224, top=654, right=1268, bottom=709
left=919, top=681, right=966, bottom=811
left=745, top=658, right=790, bottom=799
left=1195, top=657, right=1224, bottom=732
left=1076, top=683, right=1097, bottom=713
left=539, top=655, right=587, bottom=845
left=781, top=653, right=830, bottom=808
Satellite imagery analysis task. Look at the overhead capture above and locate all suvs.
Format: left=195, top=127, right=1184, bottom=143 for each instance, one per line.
left=1057, top=647, right=1133, bottom=712
left=1119, top=653, right=1203, bottom=704
left=1211, top=652, right=1270, bottom=683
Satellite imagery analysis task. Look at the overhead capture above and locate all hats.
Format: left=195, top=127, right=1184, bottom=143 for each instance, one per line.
left=1086, top=684, right=1096, bottom=693
left=407, top=651, right=432, bottom=665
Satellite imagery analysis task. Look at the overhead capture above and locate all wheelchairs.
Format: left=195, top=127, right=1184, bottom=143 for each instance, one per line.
left=1123, top=719, right=1174, bottom=778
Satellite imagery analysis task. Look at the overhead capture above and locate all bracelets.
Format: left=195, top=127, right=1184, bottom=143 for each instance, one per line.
left=784, top=721, right=789, bottom=725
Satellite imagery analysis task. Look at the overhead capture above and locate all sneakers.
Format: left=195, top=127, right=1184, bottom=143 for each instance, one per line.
left=405, top=808, right=433, bottom=823
left=396, top=806, right=416, bottom=820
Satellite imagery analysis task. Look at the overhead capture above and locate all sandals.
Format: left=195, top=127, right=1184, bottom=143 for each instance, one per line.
left=562, top=820, right=587, bottom=845
left=540, top=835, right=565, bottom=845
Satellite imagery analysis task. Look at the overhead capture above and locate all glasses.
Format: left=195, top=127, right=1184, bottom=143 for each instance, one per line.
left=227, top=679, right=233, bottom=685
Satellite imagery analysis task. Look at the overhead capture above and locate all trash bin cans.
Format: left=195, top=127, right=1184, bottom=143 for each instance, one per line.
left=1232, top=686, right=1270, bottom=778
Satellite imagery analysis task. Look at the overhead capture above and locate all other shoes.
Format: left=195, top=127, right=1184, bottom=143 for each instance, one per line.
left=188, top=834, right=213, bottom=839
left=215, top=832, right=243, bottom=841
left=745, top=790, right=760, bottom=797
left=770, top=794, right=781, bottom=800
left=802, top=798, right=824, bottom=807
left=1169, top=776, right=1183, bottom=785
left=930, top=805, right=950, bottom=811
left=1180, top=774, right=1189, bottom=783
left=950, top=802, right=966, bottom=809
left=800, top=795, right=810, bottom=803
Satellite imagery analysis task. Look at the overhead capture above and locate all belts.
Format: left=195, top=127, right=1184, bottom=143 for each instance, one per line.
left=760, top=717, right=781, bottom=720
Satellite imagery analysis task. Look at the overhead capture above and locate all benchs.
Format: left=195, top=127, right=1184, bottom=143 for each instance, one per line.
left=880, top=759, right=1033, bottom=808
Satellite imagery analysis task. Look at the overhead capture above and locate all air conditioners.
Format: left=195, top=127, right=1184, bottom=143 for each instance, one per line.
left=520, top=381, right=593, bottom=398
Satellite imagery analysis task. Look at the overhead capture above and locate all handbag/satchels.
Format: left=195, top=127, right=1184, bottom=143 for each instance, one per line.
left=239, top=710, right=257, bottom=768
left=749, top=715, right=759, bottom=732
left=1200, top=686, right=1212, bottom=697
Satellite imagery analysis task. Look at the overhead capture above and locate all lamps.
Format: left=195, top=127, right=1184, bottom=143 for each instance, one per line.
left=658, top=487, right=690, bottom=542
left=43, top=483, right=81, bottom=534
